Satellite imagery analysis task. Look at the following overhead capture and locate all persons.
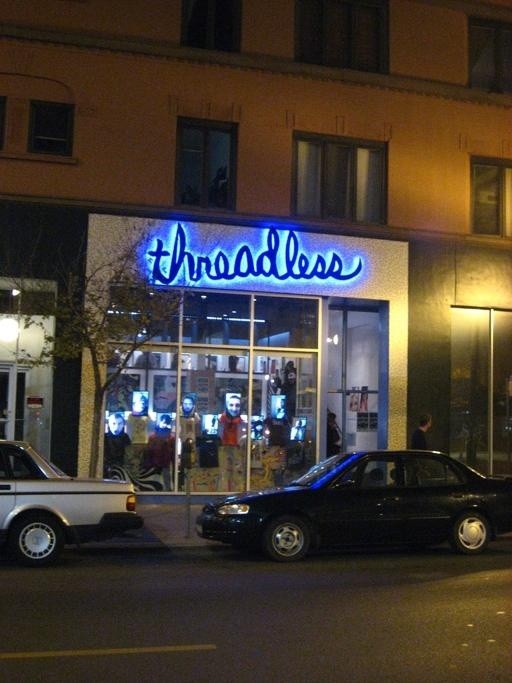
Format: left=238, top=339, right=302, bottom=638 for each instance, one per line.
left=126, top=390, right=153, bottom=449
left=326, top=413, right=341, bottom=457
left=348, top=386, right=359, bottom=411
left=100, top=412, right=133, bottom=443
left=171, top=393, right=202, bottom=468
left=359, top=386, right=370, bottom=412
left=147, top=410, right=177, bottom=466
left=214, top=395, right=246, bottom=449
left=410, top=411, right=434, bottom=449
left=259, top=395, right=296, bottom=462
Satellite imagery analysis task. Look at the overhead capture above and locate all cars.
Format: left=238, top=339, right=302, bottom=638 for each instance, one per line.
left=0, top=433, right=144, bottom=567
left=194, top=448, right=512, bottom=563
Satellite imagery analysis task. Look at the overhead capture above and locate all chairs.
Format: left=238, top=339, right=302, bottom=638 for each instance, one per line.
left=370, top=469, right=384, bottom=481
left=389, top=469, right=398, bottom=486
